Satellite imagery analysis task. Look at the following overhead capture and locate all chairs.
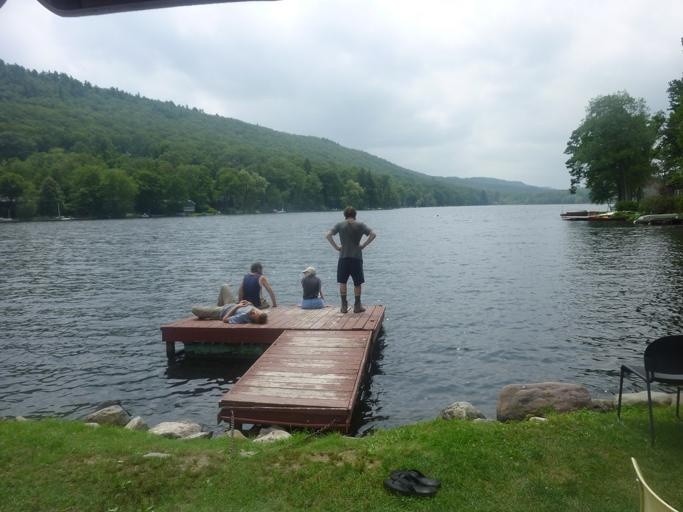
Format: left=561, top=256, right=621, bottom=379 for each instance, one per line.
left=617, top=334, right=683, bottom=442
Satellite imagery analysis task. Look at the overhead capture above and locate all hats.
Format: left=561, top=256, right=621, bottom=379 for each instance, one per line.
left=302, top=267, right=316, bottom=274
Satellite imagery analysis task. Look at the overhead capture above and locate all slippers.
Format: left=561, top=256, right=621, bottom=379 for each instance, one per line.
left=382, top=477, right=437, bottom=497
left=389, top=469, right=440, bottom=489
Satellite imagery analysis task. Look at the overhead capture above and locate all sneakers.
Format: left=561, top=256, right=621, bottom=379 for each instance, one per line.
left=340, top=305, right=347, bottom=313
left=354, top=303, right=365, bottom=313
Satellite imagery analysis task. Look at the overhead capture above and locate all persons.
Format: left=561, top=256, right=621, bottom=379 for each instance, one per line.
left=191, top=284, right=267, bottom=325
left=237, top=263, right=277, bottom=310
left=300, top=266, right=324, bottom=309
left=325, top=207, right=375, bottom=313
